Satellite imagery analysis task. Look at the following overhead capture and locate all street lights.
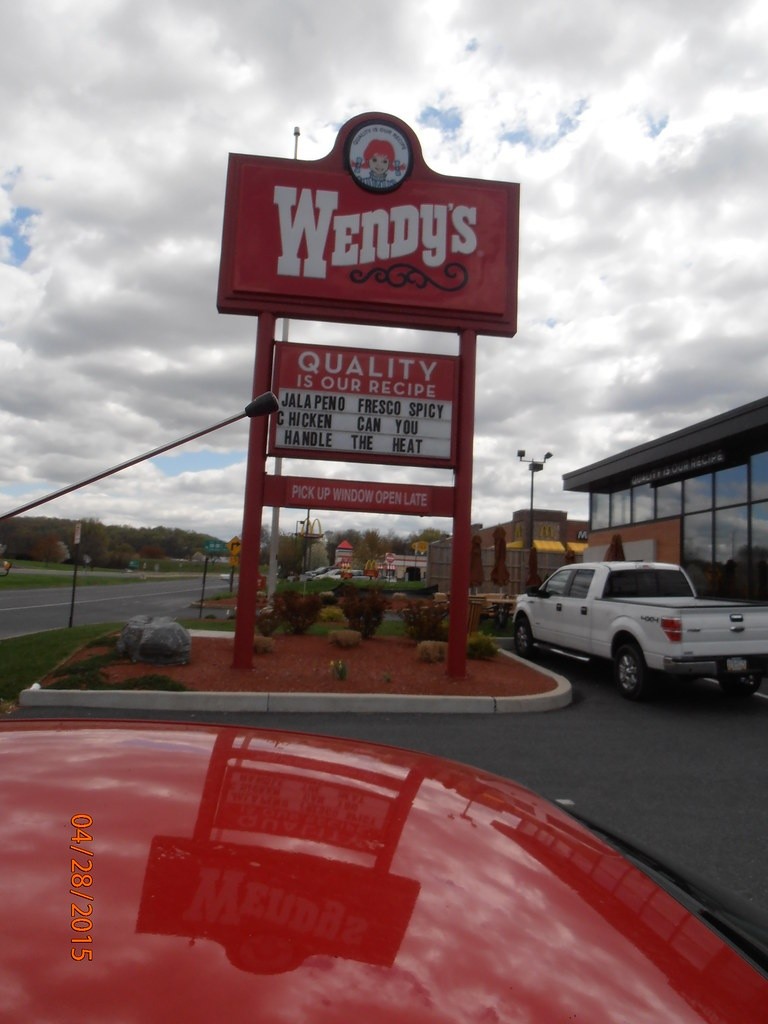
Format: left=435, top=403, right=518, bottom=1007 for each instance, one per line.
left=517, top=450, right=550, bottom=550
left=292, top=520, right=304, bottom=574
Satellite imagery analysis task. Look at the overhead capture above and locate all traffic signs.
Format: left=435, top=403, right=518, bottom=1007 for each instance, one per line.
left=203, top=539, right=228, bottom=553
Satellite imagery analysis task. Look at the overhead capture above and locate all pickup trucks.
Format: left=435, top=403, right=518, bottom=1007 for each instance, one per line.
left=511, top=561, right=768, bottom=700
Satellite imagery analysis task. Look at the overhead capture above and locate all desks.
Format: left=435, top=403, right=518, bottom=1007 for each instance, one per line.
left=487, top=599, right=516, bottom=632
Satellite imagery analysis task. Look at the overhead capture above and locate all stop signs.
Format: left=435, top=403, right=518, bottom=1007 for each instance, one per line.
left=386, top=551, right=396, bottom=565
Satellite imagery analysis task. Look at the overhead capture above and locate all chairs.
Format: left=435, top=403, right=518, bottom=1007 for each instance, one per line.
left=434, top=592, right=449, bottom=611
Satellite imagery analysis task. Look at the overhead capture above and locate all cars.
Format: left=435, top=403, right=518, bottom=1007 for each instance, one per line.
left=306, top=565, right=395, bottom=586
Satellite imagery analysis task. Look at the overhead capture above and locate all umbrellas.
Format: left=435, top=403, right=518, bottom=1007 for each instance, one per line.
left=604, top=534, right=625, bottom=561
left=468, top=534, right=485, bottom=596
left=491, top=523, right=510, bottom=594
left=565, top=550, right=575, bottom=565
left=526, top=546, right=541, bottom=586
